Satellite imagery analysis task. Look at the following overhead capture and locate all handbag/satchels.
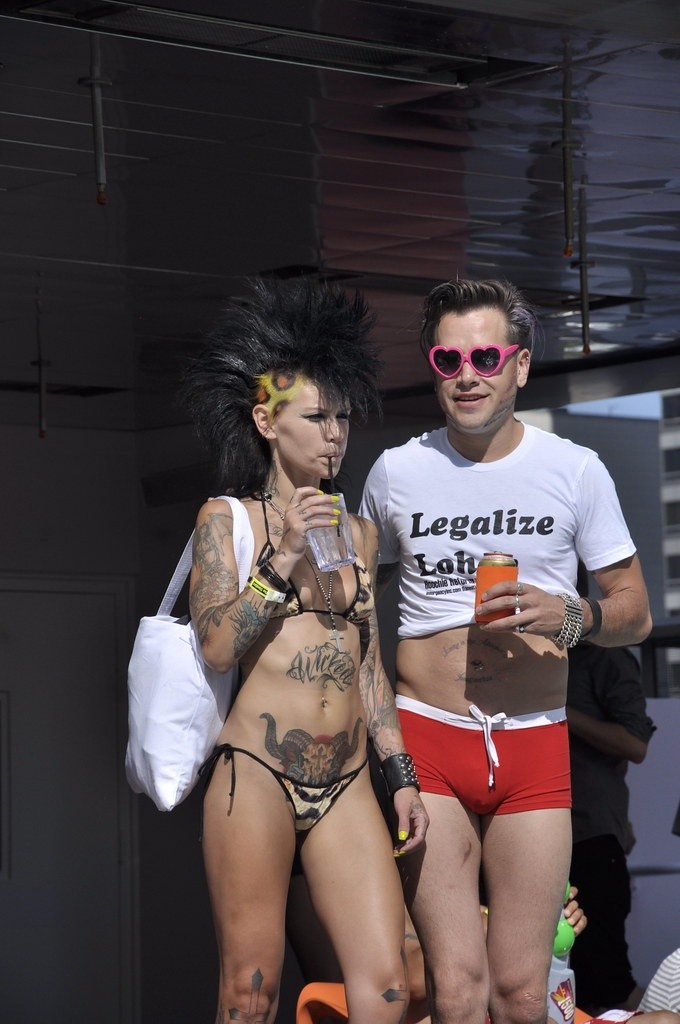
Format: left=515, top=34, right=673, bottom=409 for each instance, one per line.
left=123, top=495, right=255, bottom=813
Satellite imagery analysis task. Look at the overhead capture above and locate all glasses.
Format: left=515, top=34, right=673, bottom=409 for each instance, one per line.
left=428, top=344, right=519, bottom=379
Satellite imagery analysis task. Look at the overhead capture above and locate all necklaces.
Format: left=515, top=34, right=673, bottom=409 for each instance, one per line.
left=261, top=484, right=346, bottom=653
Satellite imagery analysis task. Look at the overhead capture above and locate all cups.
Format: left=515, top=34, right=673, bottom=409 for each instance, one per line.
left=306, top=492, right=355, bottom=572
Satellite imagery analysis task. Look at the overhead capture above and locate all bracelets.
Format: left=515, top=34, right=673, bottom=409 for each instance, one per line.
left=580, top=596, right=602, bottom=643
left=549, top=591, right=584, bottom=648
left=378, top=752, right=420, bottom=801
left=248, top=559, right=289, bottom=604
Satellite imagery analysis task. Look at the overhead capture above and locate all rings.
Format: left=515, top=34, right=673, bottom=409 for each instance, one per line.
left=515, top=582, right=523, bottom=607
left=518, top=624, right=525, bottom=633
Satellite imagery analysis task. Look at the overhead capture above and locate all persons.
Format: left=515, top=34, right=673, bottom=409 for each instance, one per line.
left=564, top=558, right=658, bottom=1018
left=628, top=948, right=680, bottom=1024
left=355, top=278, right=654, bottom=1024
left=565, top=555, right=640, bottom=1024
left=190, top=271, right=429, bottom=1023
